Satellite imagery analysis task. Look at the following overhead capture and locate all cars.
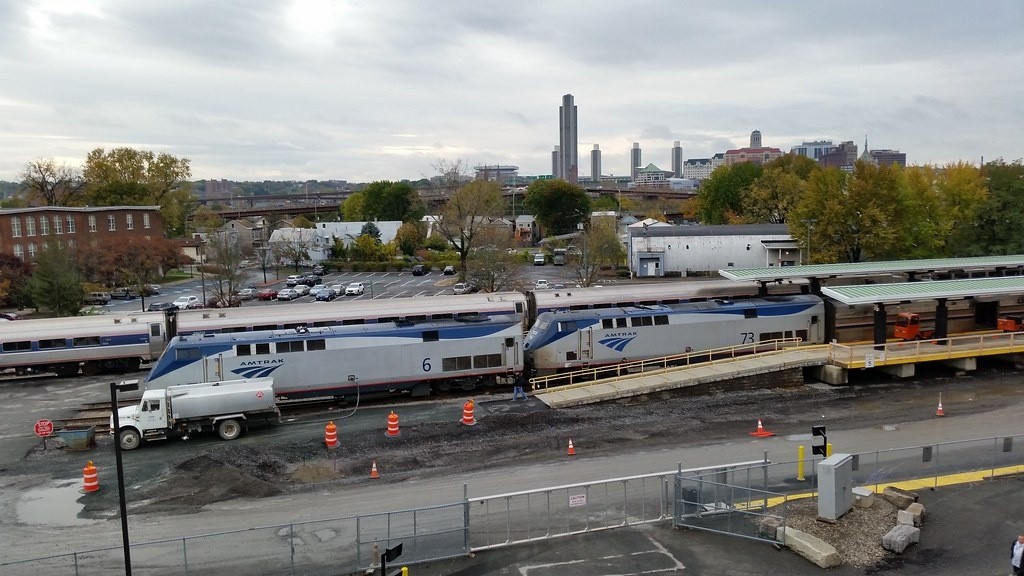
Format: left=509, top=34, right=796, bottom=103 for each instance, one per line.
left=0, top=312, right=23, bottom=321
left=506, top=248, right=517, bottom=254
left=216, top=266, right=364, bottom=308
left=172, top=296, right=198, bottom=310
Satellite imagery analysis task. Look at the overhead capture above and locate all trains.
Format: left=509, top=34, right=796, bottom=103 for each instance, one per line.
left=148, top=272, right=1024, bottom=399
left=0, top=263, right=1024, bottom=381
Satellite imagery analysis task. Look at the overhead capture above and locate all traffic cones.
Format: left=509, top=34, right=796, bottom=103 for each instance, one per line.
left=369, top=459, right=381, bottom=479
left=757, top=419, right=765, bottom=434
left=567, top=438, right=576, bottom=455
left=935, top=401, right=943, bottom=415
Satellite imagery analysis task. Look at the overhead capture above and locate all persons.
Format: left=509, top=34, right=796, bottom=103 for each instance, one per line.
left=620, top=358, right=630, bottom=375
left=511, top=371, right=528, bottom=401
left=1008, top=535, right=1024, bottom=576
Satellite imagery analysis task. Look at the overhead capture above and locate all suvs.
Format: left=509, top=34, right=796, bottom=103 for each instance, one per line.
left=412, top=265, right=473, bottom=295
left=148, top=302, right=180, bottom=315
left=84, top=292, right=111, bottom=304
left=534, top=254, right=565, bottom=290
left=109, top=284, right=160, bottom=301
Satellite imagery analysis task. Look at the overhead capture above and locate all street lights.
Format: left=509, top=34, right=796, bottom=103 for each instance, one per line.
left=110, top=379, right=139, bottom=576
left=200, top=241, right=207, bottom=306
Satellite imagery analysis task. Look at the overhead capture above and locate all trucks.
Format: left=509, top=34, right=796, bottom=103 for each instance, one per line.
left=109, top=377, right=283, bottom=450
left=998, top=315, right=1024, bottom=333
left=894, top=313, right=935, bottom=341
